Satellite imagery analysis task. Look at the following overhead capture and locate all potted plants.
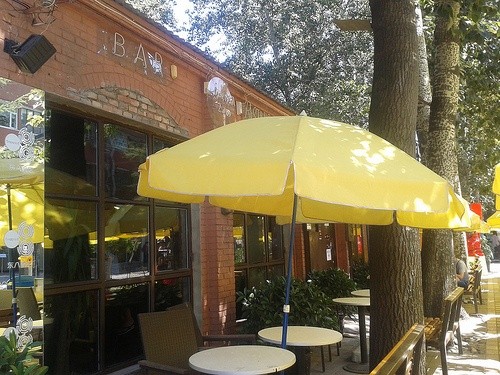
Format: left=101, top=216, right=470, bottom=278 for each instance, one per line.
left=235, top=275, right=342, bottom=375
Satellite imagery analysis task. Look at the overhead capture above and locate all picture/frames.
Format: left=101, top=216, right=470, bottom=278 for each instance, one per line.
left=325, top=248, right=332, bottom=262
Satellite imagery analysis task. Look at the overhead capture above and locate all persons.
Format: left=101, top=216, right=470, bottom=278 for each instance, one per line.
left=493, top=231, right=498, bottom=258
left=154, top=278, right=178, bottom=311
left=455, top=258, right=469, bottom=303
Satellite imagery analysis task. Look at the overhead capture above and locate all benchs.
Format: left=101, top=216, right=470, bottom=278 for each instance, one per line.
left=423, top=287, right=465, bottom=375
left=464, top=266, right=483, bottom=313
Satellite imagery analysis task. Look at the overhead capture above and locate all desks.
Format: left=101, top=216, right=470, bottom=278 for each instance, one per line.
left=351, top=288, right=370, bottom=296
left=332, top=298, right=371, bottom=373
left=256, top=327, right=343, bottom=375
left=33, top=320, right=44, bottom=329
left=0, top=328, right=12, bottom=337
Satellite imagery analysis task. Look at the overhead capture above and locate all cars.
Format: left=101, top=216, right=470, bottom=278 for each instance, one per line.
left=5, top=274, right=36, bottom=290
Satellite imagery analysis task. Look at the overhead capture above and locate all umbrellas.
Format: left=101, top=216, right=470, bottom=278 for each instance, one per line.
left=453, top=162, right=500, bottom=235
left=137, top=110, right=470, bottom=350
left=0, top=158, right=362, bottom=329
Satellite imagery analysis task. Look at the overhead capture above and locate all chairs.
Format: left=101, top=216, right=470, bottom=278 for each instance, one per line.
left=0, top=278, right=44, bottom=366
left=368, top=322, right=425, bottom=375
left=137, top=301, right=257, bottom=375
